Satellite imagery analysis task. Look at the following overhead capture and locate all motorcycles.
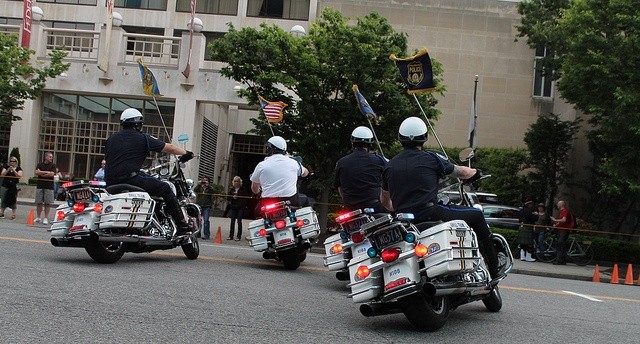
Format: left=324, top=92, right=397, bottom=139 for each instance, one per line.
left=345, top=147, right=514, bottom=332
left=322, top=206, right=390, bottom=281
left=245, top=155, right=321, bottom=270
left=46, top=132, right=203, bottom=263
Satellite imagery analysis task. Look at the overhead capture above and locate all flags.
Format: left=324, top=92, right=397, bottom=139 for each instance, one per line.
left=137, top=59, right=163, bottom=98
left=258, top=95, right=288, bottom=124
left=353, top=85, right=380, bottom=126
left=391, top=48, right=436, bottom=96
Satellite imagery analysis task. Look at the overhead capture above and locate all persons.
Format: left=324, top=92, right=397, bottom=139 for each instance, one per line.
left=102, top=108, right=196, bottom=234
left=0, top=156, right=24, bottom=219
left=334, top=126, right=395, bottom=218
left=550, top=200, right=571, bottom=265
left=379, top=116, right=512, bottom=281
left=516, top=197, right=540, bottom=262
left=193, top=176, right=218, bottom=240
left=533, top=203, right=550, bottom=262
left=54, top=167, right=63, bottom=200
left=95, top=160, right=105, bottom=180
left=227, top=175, right=248, bottom=241
left=34, top=152, right=57, bottom=224
left=249, top=134, right=309, bottom=259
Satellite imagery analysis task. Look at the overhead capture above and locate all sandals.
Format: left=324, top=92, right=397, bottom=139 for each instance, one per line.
left=10, top=216, right=16, bottom=220
left=0, top=214, right=5, bottom=219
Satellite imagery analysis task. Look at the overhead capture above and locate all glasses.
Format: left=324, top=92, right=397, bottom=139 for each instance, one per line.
left=49, top=156, right=53, bottom=158
left=10, top=160, right=16, bottom=162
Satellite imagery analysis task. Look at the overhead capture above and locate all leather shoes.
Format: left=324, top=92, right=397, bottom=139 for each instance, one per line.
left=234, top=237, right=240, bottom=241
left=226, top=236, right=233, bottom=240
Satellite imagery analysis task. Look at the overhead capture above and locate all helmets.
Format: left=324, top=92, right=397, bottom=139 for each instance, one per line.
left=263, top=136, right=287, bottom=156
left=120, top=108, right=145, bottom=125
left=398, top=117, right=428, bottom=143
left=350, top=126, right=375, bottom=143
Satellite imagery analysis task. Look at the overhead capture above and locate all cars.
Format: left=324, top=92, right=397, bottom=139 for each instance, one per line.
left=478, top=203, right=524, bottom=229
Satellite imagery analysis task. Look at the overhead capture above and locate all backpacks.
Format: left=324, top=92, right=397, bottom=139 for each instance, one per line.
left=562, top=209, right=576, bottom=229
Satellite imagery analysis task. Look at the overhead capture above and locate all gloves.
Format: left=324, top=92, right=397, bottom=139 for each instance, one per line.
left=183, top=150, right=194, bottom=159
left=467, top=169, right=485, bottom=182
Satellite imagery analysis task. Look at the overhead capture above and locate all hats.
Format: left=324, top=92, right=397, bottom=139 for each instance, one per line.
left=524, top=198, right=533, bottom=205
left=537, top=202, right=547, bottom=209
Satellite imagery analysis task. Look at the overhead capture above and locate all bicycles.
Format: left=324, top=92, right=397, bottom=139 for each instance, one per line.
left=534, top=221, right=594, bottom=266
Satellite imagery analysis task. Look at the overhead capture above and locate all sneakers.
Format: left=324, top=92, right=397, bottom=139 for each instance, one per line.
left=201, top=235, right=210, bottom=239
left=562, top=260, right=566, bottom=265
left=43, top=219, right=48, bottom=224
left=34, top=218, right=41, bottom=223
left=552, top=260, right=563, bottom=265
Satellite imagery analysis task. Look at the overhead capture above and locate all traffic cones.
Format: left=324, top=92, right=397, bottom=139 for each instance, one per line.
left=26, top=207, right=36, bottom=224
left=212, top=225, right=223, bottom=244
left=592, top=263, right=600, bottom=282
left=609, top=263, right=620, bottom=284
left=636, top=273, right=640, bottom=286
left=623, top=263, right=634, bottom=285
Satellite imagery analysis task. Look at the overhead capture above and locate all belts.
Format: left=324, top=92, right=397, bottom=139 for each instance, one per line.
left=106, top=172, right=138, bottom=183
left=394, top=201, right=434, bottom=216
left=352, top=197, right=379, bottom=207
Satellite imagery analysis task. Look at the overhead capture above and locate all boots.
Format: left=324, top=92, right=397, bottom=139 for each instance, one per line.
left=163, top=192, right=194, bottom=233
left=520, top=249, right=526, bottom=261
left=478, top=227, right=507, bottom=279
left=525, top=249, right=536, bottom=262
